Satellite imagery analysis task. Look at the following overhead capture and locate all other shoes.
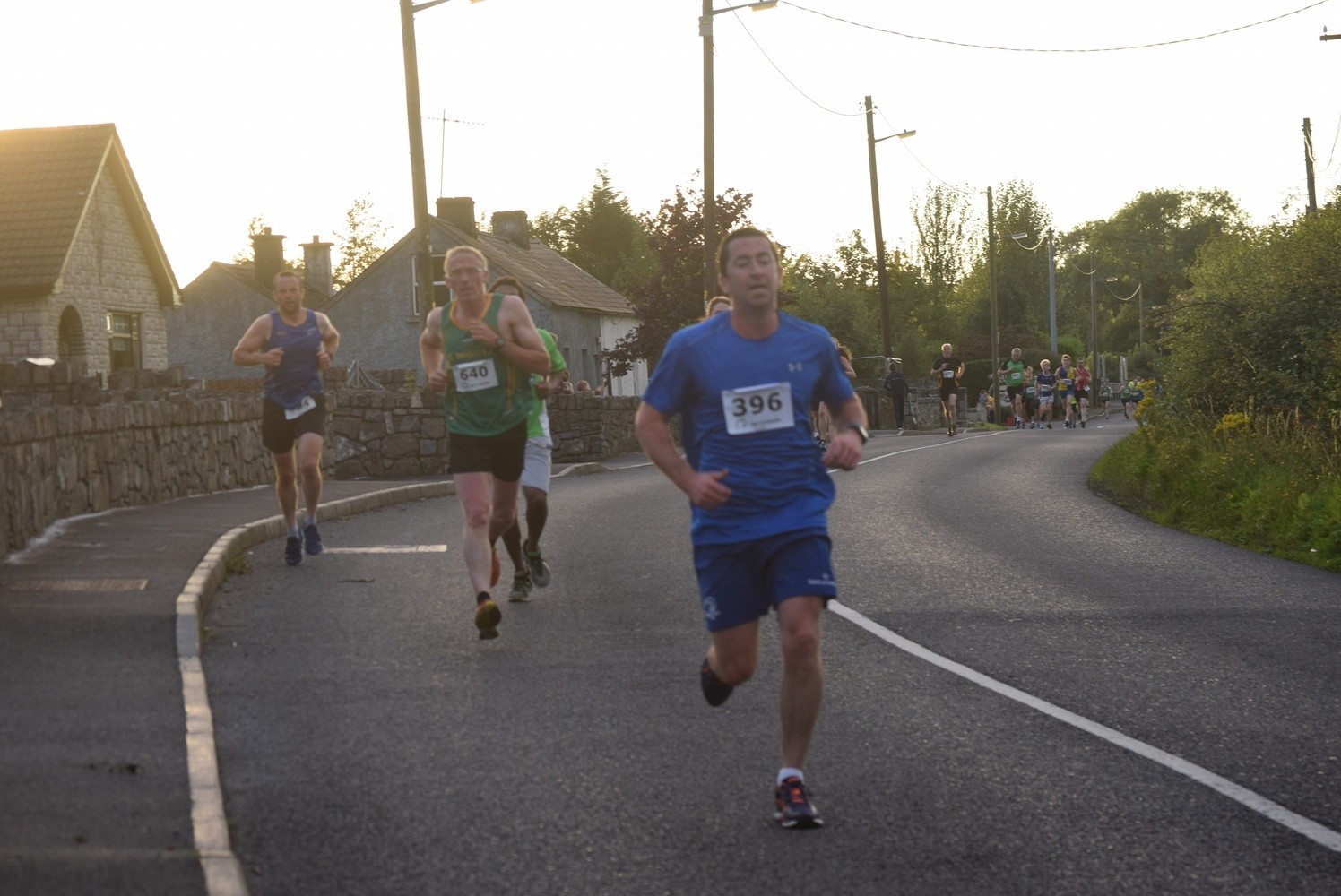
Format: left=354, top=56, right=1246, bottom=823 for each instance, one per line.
left=819, top=442, right=827, bottom=450
left=953, top=428, right=956, bottom=434
left=947, top=429, right=953, bottom=436
left=1015, top=416, right=1086, bottom=428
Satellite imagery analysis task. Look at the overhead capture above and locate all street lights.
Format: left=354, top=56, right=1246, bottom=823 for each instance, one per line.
left=1139, top=303, right=1169, bottom=378
left=1091, top=276, right=1119, bottom=410
left=1048, top=249, right=1080, bottom=358
left=865, top=94, right=917, bottom=353
left=983, top=187, right=1028, bottom=424
left=699, top=0, right=779, bottom=301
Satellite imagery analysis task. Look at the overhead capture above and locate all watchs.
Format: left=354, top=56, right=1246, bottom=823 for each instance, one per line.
left=846, top=422, right=869, bottom=446
left=494, top=337, right=505, bottom=351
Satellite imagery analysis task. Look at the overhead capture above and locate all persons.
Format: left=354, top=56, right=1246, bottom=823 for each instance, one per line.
left=231, top=271, right=339, bottom=564
left=931, top=343, right=964, bottom=436
left=632, top=228, right=868, bottom=828
left=981, top=347, right=1144, bottom=429
left=488, top=276, right=606, bottom=602
left=882, top=364, right=910, bottom=436
left=418, top=244, right=553, bottom=641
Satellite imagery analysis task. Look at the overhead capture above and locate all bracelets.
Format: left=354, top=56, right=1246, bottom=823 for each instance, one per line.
left=327, top=351, right=333, bottom=361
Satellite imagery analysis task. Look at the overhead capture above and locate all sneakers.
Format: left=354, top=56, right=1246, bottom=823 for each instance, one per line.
left=520, top=540, right=552, bottom=587
left=301, top=519, right=323, bottom=555
left=772, top=775, right=824, bottom=830
left=474, top=600, right=502, bottom=640
left=490, top=545, right=500, bottom=586
left=284, top=530, right=303, bottom=565
left=507, top=567, right=533, bottom=602
left=699, top=657, right=735, bottom=708
